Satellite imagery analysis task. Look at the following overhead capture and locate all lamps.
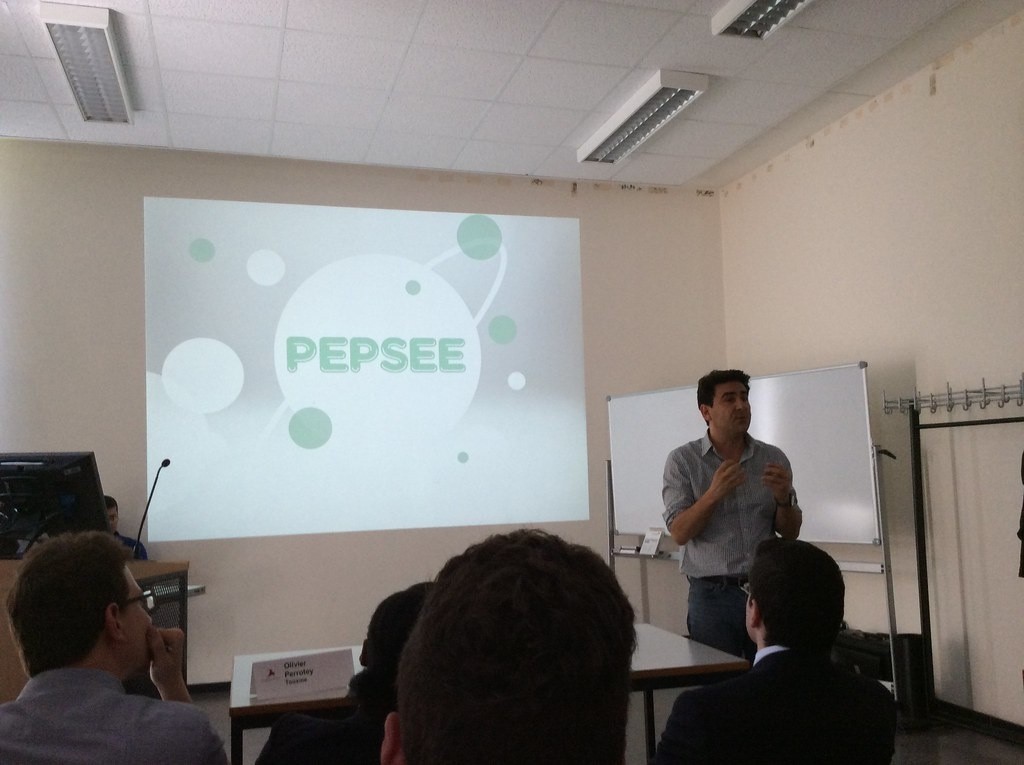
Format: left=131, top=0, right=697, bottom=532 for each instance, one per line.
left=41, top=5, right=138, bottom=125
left=711, top=0, right=812, bottom=41
left=575, top=71, right=705, bottom=168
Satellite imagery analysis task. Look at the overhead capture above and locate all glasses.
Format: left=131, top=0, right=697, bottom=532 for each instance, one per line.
left=740, top=584, right=751, bottom=598
left=120, top=590, right=157, bottom=613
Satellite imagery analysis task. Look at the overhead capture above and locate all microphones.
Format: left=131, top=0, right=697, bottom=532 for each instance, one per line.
left=134, top=459, right=170, bottom=558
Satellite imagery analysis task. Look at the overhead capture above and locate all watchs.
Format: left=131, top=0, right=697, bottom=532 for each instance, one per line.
left=774, top=494, right=795, bottom=507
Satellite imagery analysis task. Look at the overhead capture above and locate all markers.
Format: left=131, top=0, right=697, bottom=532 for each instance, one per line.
left=622, top=546, right=641, bottom=550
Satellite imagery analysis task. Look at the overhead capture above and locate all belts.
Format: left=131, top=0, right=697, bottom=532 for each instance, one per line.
left=703, top=576, right=748, bottom=587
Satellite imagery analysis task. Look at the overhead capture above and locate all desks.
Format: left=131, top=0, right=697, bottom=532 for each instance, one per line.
left=229, top=621, right=748, bottom=765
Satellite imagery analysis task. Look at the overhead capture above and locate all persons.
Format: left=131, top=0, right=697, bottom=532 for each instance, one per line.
left=380, top=528, right=636, bottom=765
left=662, top=370, right=802, bottom=663
left=0, top=530, right=228, bottom=765
left=104, top=495, right=147, bottom=560
left=255, top=581, right=434, bottom=765
left=656, top=541, right=897, bottom=765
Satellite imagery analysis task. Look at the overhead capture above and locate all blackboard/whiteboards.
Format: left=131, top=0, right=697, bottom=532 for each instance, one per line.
left=605, top=360, right=881, bottom=546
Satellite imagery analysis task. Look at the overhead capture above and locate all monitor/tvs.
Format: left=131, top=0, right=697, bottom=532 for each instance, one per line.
left=0, top=451, right=112, bottom=560
